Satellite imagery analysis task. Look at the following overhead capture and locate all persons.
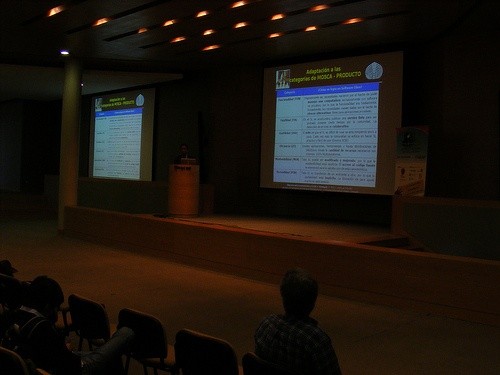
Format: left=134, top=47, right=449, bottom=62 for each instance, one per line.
left=0, top=260, right=32, bottom=308
left=174, top=143, right=193, bottom=165
left=0, top=275, right=134, bottom=375
left=254, top=269, right=341, bottom=375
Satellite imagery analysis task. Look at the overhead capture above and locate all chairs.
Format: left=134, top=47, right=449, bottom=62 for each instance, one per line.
left=0, top=273, right=69, bottom=327
left=174, top=328, right=244, bottom=375
left=117, top=308, right=176, bottom=375
left=68, top=294, right=117, bottom=352
left=0, top=346, right=52, bottom=375
left=242, top=353, right=286, bottom=375
